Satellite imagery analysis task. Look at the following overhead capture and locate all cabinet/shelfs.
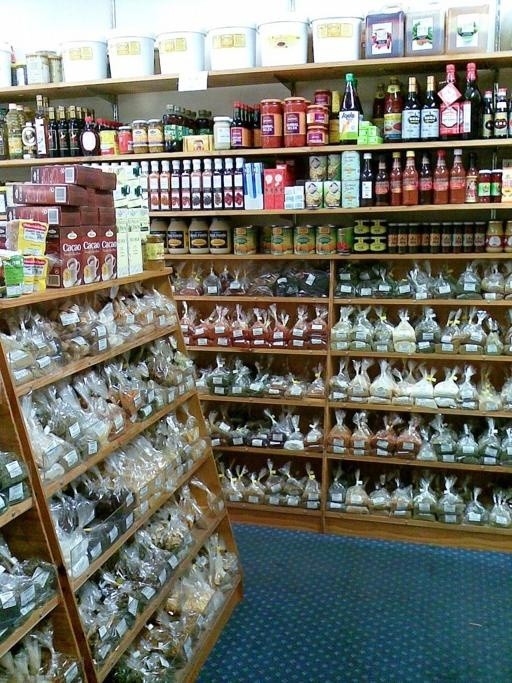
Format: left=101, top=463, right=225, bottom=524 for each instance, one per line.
left=0, top=267, right=244, bottom=682
left=0, top=376, right=88, bottom=683
left=0, top=52, right=512, bottom=551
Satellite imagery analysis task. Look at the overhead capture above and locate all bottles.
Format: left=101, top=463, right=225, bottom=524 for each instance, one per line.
left=359, top=147, right=478, bottom=203
left=325, top=62, right=511, bottom=139
left=229, top=100, right=262, bottom=149
left=76, top=155, right=244, bottom=209
left=164, top=101, right=214, bottom=148
left=0, top=94, right=129, bottom=161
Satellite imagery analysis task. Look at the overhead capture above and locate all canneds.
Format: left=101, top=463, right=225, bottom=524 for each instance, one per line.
left=21, top=126, right=38, bottom=159
left=150, top=216, right=512, bottom=255
left=491, top=169, right=502, bottom=203
left=145, top=233, right=164, bottom=270
left=11, top=50, right=62, bottom=86
left=213, top=116, right=231, bottom=150
left=98, top=119, right=165, bottom=156
left=259, top=89, right=330, bottom=149
left=478, top=169, right=491, bottom=203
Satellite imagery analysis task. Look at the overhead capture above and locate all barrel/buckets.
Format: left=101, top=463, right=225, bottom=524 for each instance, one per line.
left=61, top=38, right=107, bottom=82
left=0, top=43, right=12, bottom=84
left=364, top=1, right=512, bottom=58
left=311, top=15, right=362, bottom=64
left=156, top=31, right=209, bottom=76
left=106, top=32, right=156, bottom=79
left=206, top=25, right=258, bottom=71
left=255, top=17, right=312, bottom=70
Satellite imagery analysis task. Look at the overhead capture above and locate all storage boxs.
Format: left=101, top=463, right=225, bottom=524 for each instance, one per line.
left=446, top=4, right=494, bottom=52
left=405, top=8, right=445, bottom=56
left=364, top=8, right=405, bottom=59
